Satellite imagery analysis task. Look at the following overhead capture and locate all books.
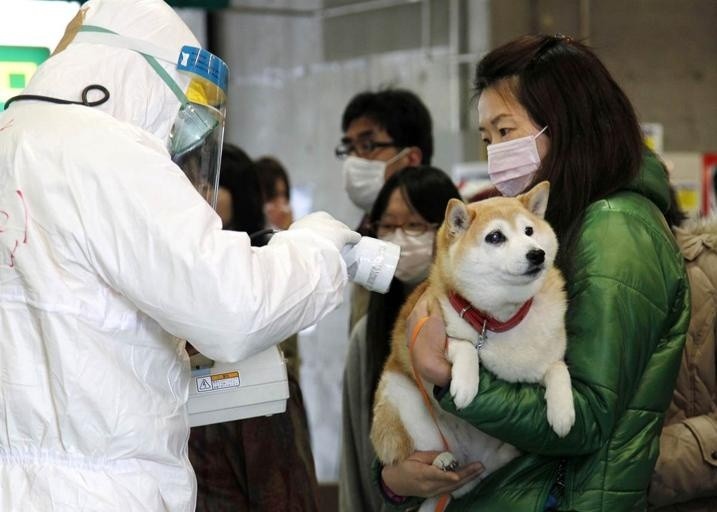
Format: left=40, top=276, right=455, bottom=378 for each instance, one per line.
left=187, top=352, right=290, bottom=426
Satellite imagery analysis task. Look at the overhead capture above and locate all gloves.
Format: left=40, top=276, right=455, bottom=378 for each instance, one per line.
left=287, top=211, right=361, bottom=252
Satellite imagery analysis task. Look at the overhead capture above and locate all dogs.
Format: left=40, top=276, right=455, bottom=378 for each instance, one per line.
left=368, top=178, right=575, bottom=499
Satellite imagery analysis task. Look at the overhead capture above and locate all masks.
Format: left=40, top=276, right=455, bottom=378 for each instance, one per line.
left=344, top=146, right=411, bottom=213
left=487, top=123, right=548, bottom=198
left=379, top=231, right=438, bottom=285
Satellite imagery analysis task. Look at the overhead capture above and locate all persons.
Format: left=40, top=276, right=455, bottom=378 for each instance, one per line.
left=1, top=0, right=362, bottom=512
left=372, top=30, right=691, bottom=511
left=206, top=142, right=269, bottom=246
left=253, top=156, right=294, bottom=229
left=653, top=164, right=717, bottom=512
left=335, top=88, right=435, bottom=327
left=336, top=164, right=465, bottom=512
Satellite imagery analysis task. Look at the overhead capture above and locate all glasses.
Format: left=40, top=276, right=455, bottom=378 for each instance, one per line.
left=79, top=23, right=219, bottom=164
left=335, top=142, right=404, bottom=160
